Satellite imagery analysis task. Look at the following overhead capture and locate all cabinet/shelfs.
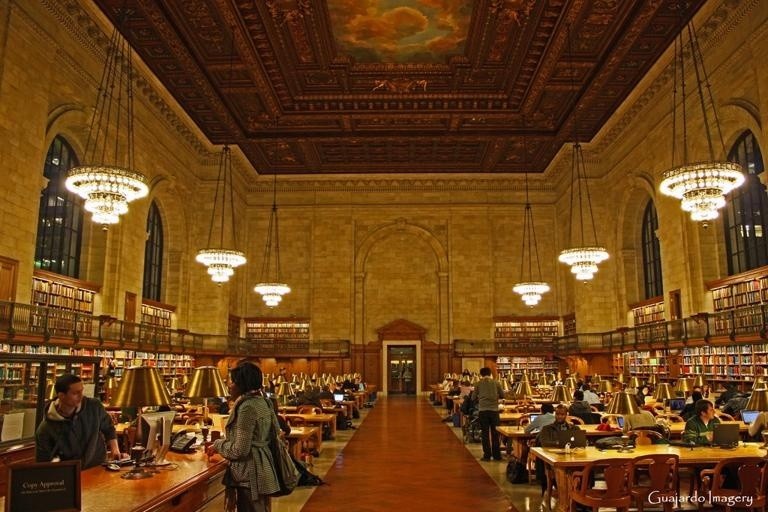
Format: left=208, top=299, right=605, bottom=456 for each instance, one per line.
left=28, top=276, right=96, bottom=341
left=628, top=272, right=768, bottom=344
left=140, top=299, right=176, bottom=346
left=613, top=344, right=768, bottom=390
left=1, top=341, right=198, bottom=404
left=494, top=312, right=576, bottom=351
left=244, top=318, right=309, bottom=353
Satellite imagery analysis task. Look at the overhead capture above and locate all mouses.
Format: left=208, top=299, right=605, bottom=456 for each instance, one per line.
left=107, top=464, right=121, bottom=471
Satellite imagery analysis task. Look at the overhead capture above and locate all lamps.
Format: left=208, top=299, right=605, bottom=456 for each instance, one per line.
left=253, top=163, right=291, bottom=309
left=512, top=204, right=550, bottom=307
left=556, top=143, right=608, bottom=283
left=657, top=0, right=748, bottom=225
left=195, top=146, right=247, bottom=284
left=64, top=2, right=149, bottom=232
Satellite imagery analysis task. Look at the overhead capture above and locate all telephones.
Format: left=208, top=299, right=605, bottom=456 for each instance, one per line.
left=168, top=429, right=197, bottom=454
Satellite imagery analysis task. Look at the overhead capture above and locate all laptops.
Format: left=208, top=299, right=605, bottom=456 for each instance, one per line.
left=712, top=423, right=740, bottom=449
left=644, top=395, right=654, bottom=406
left=616, top=417, right=624, bottom=430
left=558, top=429, right=586, bottom=448
left=333, top=393, right=344, bottom=402
left=530, top=414, right=539, bottom=424
left=669, top=398, right=685, bottom=413
left=739, top=409, right=761, bottom=424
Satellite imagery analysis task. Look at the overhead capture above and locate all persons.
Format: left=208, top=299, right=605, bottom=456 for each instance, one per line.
left=208, top=363, right=280, bottom=512
left=402, top=367, right=413, bottom=395
left=35, top=374, right=121, bottom=464
left=443, top=369, right=768, bottom=495
left=272, top=375, right=367, bottom=435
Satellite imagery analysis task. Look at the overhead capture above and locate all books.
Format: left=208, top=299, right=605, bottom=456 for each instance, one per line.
left=495, top=276, right=768, bottom=382
left=0, top=271, right=309, bottom=401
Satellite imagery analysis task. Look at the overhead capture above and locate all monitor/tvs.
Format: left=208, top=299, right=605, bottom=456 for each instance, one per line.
left=140, top=409, right=175, bottom=467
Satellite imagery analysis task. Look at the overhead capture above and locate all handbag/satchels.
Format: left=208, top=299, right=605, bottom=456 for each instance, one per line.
left=506, top=455, right=528, bottom=484
left=270, top=430, right=302, bottom=498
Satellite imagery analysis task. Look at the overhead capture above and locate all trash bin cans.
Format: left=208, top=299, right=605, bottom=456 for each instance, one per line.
left=430, top=393, right=436, bottom=401
left=370, top=392, right=376, bottom=401
left=453, top=414, right=460, bottom=427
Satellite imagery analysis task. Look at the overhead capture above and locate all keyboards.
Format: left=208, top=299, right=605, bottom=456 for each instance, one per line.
left=102, top=458, right=155, bottom=468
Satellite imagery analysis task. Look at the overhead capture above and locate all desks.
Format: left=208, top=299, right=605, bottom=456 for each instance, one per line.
left=172, top=384, right=768, bottom=511
